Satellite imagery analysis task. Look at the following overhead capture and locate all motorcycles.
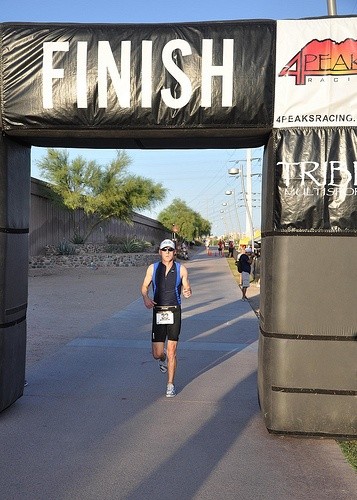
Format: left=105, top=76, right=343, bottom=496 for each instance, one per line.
left=180, top=242, right=190, bottom=260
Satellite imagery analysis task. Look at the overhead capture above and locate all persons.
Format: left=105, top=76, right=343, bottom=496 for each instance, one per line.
left=142, top=239, right=192, bottom=398
left=179, top=239, right=255, bottom=302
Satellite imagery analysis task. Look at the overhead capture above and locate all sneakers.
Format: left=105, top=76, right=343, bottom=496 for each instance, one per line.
left=159, top=348, right=168, bottom=374
left=165, top=384, right=176, bottom=397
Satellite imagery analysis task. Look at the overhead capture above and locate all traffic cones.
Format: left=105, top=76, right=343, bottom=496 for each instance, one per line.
left=221, top=249, right=224, bottom=257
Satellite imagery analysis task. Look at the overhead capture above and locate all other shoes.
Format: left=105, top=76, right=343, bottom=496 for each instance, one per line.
left=242, top=296, right=250, bottom=302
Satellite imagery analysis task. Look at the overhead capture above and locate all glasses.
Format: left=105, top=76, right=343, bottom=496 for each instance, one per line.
left=161, top=247, right=175, bottom=251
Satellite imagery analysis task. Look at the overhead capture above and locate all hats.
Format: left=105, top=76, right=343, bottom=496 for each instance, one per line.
left=245, top=248, right=253, bottom=253
left=159, top=239, right=175, bottom=249
